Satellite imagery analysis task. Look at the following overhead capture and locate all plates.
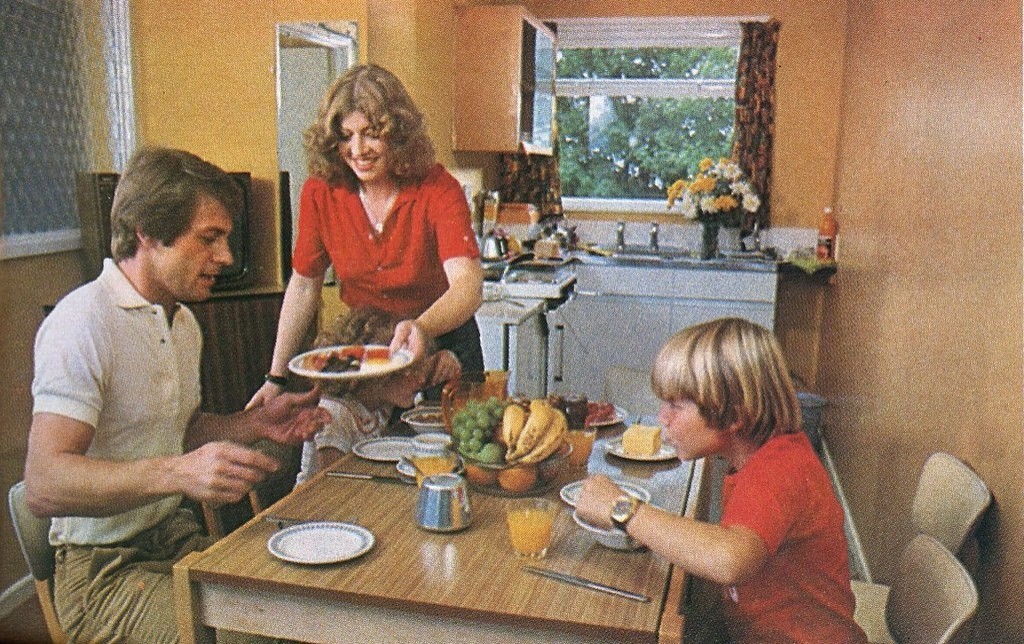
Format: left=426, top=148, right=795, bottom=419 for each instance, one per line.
left=287, top=344, right=417, bottom=377
left=563, top=425, right=597, bottom=467
left=560, top=478, right=651, bottom=512
left=353, top=438, right=415, bottom=460
left=606, top=436, right=678, bottom=460
left=394, top=456, right=467, bottom=477
left=268, top=520, right=376, bottom=565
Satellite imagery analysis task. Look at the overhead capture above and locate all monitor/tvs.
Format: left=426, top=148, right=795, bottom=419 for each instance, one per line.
left=76, top=169, right=253, bottom=292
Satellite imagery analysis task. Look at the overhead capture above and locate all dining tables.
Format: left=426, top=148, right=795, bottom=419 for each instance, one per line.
left=172, top=407, right=716, bottom=643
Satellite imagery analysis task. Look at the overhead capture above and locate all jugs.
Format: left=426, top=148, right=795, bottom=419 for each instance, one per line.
left=477, top=230, right=509, bottom=262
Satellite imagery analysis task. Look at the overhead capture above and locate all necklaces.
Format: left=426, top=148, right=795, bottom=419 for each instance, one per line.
left=360, top=186, right=396, bottom=234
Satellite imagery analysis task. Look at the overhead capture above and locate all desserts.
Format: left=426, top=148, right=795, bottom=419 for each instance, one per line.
left=623, top=425, right=662, bottom=455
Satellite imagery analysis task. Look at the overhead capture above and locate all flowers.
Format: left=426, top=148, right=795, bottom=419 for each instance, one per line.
left=664, top=154, right=763, bottom=221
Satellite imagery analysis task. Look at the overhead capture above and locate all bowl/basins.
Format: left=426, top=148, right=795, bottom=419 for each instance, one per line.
left=401, top=406, right=455, bottom=433
left=573, top=507, right=643, bottom=551
left=413, top=434, right=452, bottom=459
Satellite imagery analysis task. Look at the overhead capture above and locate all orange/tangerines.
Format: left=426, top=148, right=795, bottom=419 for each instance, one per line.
left=467, top=465, right=537, bottom=494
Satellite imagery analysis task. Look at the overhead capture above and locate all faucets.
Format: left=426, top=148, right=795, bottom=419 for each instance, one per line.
left=648, top=220, right=660, bottom=253
left=614, top=217, right=627, bottom=254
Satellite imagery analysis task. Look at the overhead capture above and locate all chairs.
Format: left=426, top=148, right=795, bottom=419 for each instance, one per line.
left=848, top=452, right=996, bottom=644
left=9, top=479, right=70, bottom=644
left=881, top=533, right=984, bottom=644
left=244, top=435, right=301, bottom=518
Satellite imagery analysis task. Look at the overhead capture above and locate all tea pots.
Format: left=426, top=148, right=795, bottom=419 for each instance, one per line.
left=444, top=369, right=495, bottom=435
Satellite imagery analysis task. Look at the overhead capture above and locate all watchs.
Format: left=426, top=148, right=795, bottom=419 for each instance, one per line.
left=611, top=494, right=645, bottom=536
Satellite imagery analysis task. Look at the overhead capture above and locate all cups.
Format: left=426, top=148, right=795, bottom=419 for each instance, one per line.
left=585, top=401, right=627, bottom=427
left=506, top=497, right=559, bottom=560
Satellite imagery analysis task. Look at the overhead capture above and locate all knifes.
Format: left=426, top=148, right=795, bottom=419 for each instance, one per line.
left=523, top=565, right=652, bottom=603
left=323, top=471, right=418, bottom=486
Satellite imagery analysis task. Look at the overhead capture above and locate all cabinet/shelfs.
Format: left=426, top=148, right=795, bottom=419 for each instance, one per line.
left=41, top=284, right=286, bottom=413
left=568, top=261, right=672, bottom=408
left=448, top=3, right=558, bottom=158
left=667, top=257, right=780, bottom=342
left=500, top=286, right=579, bottom=417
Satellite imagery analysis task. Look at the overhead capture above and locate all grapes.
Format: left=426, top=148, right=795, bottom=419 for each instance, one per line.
left=452, top=396, right=506, bottom=457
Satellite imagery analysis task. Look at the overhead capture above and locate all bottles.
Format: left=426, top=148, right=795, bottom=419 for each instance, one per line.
left=815, top=206, right=839, bottom=263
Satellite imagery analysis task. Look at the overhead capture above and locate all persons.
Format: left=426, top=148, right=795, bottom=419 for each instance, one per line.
left=240, top=63, right=485, bottom=425
left=292, top=304, right=462, bottom=493
left=574, top=319, right=870, bottom=643
left=25, top=144, right=331, bottom=644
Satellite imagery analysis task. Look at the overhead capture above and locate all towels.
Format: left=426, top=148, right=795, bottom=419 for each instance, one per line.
left=788, top=254, right=834, bottom=274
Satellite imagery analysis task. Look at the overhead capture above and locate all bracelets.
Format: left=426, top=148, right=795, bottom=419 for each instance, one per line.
left=265, top=373, right=287, bottom=387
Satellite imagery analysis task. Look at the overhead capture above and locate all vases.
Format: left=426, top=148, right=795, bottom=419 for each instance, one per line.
left=698, top=214, right=720, bottom=262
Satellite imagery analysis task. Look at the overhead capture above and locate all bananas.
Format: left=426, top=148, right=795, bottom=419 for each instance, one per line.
left=502, top=398, right=567, bottom=467
